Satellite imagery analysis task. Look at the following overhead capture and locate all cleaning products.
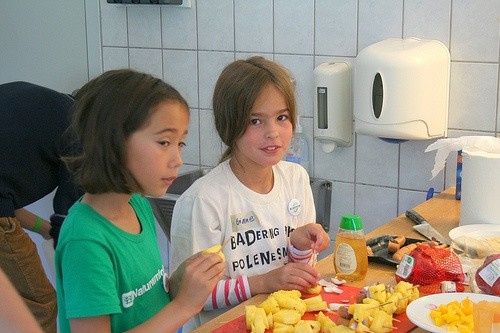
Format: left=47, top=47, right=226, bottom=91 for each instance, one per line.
left=285, top=115, right=310, bottom=179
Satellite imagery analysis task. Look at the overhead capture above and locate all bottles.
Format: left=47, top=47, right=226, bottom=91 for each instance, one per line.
left=334, top=214, right=368, bottom=281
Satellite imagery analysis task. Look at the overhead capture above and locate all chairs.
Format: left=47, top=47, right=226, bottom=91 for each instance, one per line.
left=145, top=169, right=206, bottom=244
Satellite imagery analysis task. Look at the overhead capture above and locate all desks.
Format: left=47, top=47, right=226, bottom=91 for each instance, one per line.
left=187, top=185, right=500, bottom=333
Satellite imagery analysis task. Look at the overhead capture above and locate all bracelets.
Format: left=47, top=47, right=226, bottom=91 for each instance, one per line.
left=31, top=216, right=43, bottom=232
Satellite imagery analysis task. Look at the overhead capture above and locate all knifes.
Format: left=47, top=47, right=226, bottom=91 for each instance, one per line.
left=406, top=209, right=464, bottom=253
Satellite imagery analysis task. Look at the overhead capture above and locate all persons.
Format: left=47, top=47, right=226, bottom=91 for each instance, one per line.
left=54, top=69, right=226, bottom=333
left=0, top=81, right=86, bottom=333
left=169, top=56, right=331, bottom=333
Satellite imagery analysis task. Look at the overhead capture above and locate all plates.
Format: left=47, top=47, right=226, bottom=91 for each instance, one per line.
left=366, top=235, right=450, bottom=266
left=406, top=293, right=500, bottom=333
left=448, top=224, right=500, bottom=255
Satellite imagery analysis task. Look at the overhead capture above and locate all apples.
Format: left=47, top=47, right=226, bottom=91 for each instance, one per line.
left=475, top=254, right=500, bottom=297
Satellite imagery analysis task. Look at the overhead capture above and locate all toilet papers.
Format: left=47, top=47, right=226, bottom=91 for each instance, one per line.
left=424, top=136, right=500, bottom=226
